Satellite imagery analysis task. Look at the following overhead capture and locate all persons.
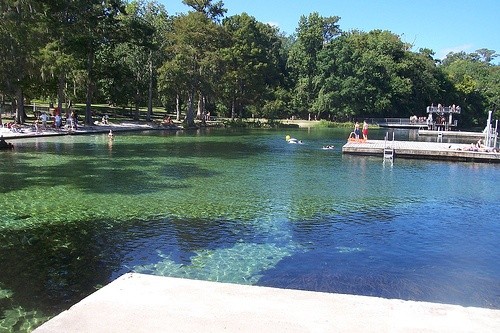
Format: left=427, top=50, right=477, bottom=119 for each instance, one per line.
left=354, top=121, right=360, bottom=141
left=428, top=103, right=460, bottom=124
left=54, top=114, right=61, bottom=129
left=40, top=112, right=47, bottom=129
left=102, top=114, right=109, bottom=124
left=410, top=114, right=417, bottom=125
left=74, top=112, right=78, bottom=129
left=362, top=120, right=368, bottom=140
